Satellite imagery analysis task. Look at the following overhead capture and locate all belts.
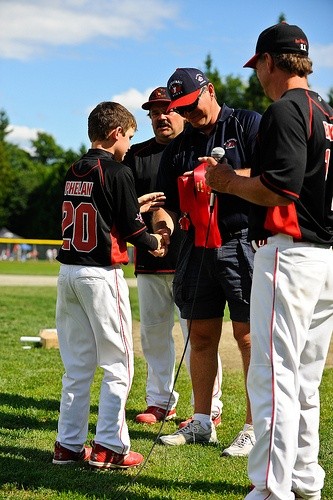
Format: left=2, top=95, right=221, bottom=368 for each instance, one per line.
left=259, top=236, right=307, bottom=247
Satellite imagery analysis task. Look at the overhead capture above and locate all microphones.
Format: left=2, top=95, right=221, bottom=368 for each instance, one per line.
left=210, top=147, right=225, bottom=210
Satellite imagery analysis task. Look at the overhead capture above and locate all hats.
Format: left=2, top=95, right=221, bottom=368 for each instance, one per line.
left=141, top=86, right=171, bottom=110
left=166, top=68, right=209, bottom=113
left=243, top=21, right=309, bottom=67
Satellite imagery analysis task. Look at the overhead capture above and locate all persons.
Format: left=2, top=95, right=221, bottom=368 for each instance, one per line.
left=194, top=20, right=333, bottom=500
left=160, top=67, right=263, bottom=459
left=122, top=86, right=225, bottom=430
left=50, top=100, right=168, bottom=470
left=0, top=243, right=38, bottom=263
left=47, top=246, right=58, bottom=263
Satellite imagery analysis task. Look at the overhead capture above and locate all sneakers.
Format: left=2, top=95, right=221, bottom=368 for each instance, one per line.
left=90, top=439, right=144, bottom=469
left=178, top=412, right=221, bottom=429
left=136, top=406, right=177, bottom=423
left=52, top=441, right=93, bottom=464
left=159, top=419, right=217, bottom=446
left=220, top=430, right=256, bottom=457
